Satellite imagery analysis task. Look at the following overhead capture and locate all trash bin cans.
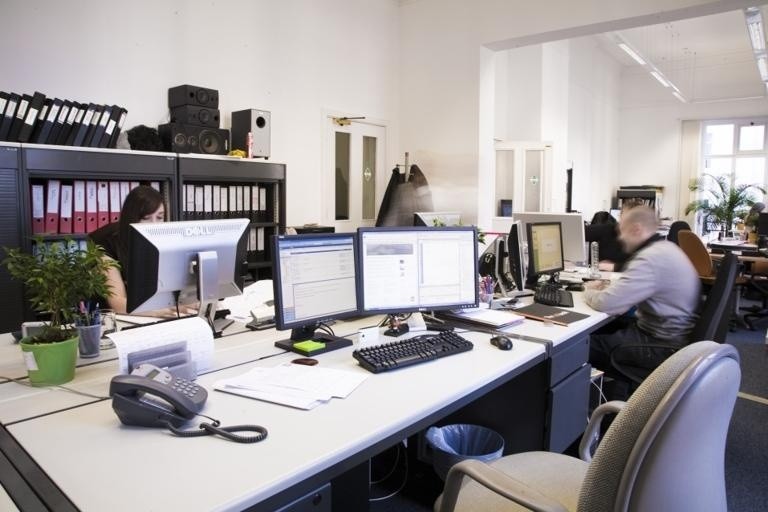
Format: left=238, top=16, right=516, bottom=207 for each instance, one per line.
left=424, top=422, right=506, bottom=481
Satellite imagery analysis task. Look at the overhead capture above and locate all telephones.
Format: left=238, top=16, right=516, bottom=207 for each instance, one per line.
left=107, top=361, right=210, bottom=431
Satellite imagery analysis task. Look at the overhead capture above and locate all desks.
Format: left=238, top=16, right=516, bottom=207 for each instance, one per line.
left=707, top=237, right=759, bottom=251
left=0, top=291, right=380, bottom=428
left=0, top=310, right=547, bottom=511
left=483, top=271, right=633, bottom=453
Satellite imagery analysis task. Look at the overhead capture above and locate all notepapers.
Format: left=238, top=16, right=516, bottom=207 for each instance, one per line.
left=293, top=340, right=325, bottom=352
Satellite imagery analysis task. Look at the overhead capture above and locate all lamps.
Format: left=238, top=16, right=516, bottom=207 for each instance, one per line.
left=744, top=6, right=768, bottom=88
left=615, top=23, right=696, bottom=104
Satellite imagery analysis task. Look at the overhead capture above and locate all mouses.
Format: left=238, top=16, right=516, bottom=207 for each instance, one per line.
left=490, top=335, right=513, bottom=350
left=566, top=282, right=581, bottom=291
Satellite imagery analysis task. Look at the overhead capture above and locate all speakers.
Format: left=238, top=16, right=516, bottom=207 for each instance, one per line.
left=160, top=85, right=230, bottom=154
left=232, top=109, right=272, bottom=159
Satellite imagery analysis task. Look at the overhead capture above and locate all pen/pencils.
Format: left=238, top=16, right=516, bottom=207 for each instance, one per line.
left=75, top=297, right=104, bottom=359
left=477, top=274, right=498, bottom=302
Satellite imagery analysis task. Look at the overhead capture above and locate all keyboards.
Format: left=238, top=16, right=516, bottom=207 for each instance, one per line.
left=533, top=284, right=574, bottom=307
left=353, top=329, right=473, bottom=372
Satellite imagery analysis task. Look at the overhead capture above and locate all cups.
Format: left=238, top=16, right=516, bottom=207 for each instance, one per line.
left=97, top=308, right=118, bottom=350
left=479, top=291, right=493, bottom=304
left=748, top=232, right=758, bottom=244
left=73, top=319, right=101, bottom=358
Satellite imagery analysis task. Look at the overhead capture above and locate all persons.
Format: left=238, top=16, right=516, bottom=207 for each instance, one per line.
left=583, top=205, right=702, bottom=371
left=92, top=184, right=202, bottom=319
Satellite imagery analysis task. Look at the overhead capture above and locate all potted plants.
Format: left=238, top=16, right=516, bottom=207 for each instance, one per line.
left=0, top=233, right=121, bottom=387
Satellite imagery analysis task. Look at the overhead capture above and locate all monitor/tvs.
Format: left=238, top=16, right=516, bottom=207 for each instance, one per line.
left=512, top=211, right=588, bottom=263
left=358, top=227, right=476, bottom=337
left=128, top=216, right=252, bottom=335
left=271, top=232, right=358, bottom=357
left=496, top=219, right=533, bottom=298
left=526, top=220, right=563, bottom=291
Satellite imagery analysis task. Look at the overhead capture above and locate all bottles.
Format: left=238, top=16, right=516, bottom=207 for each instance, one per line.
left=245, top=132, right=254, bottom=159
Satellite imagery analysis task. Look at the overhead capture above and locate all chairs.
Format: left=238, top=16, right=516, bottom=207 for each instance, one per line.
left=434, top=339, right=742, bottom=512
left=677, top=229, right=750, bottom=332
left=609, top=253, right=740, bottom=385
left=666, top=221, right=691, bottom=246
left=738, top=248, right=768, bottom=331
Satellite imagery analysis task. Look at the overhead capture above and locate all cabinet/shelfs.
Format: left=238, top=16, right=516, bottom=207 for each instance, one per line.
left=178, top=156, right=286, bottom=281
left=616, top=185, right=664, bottom=228
left=0, top=143, right=179, bottom=322
